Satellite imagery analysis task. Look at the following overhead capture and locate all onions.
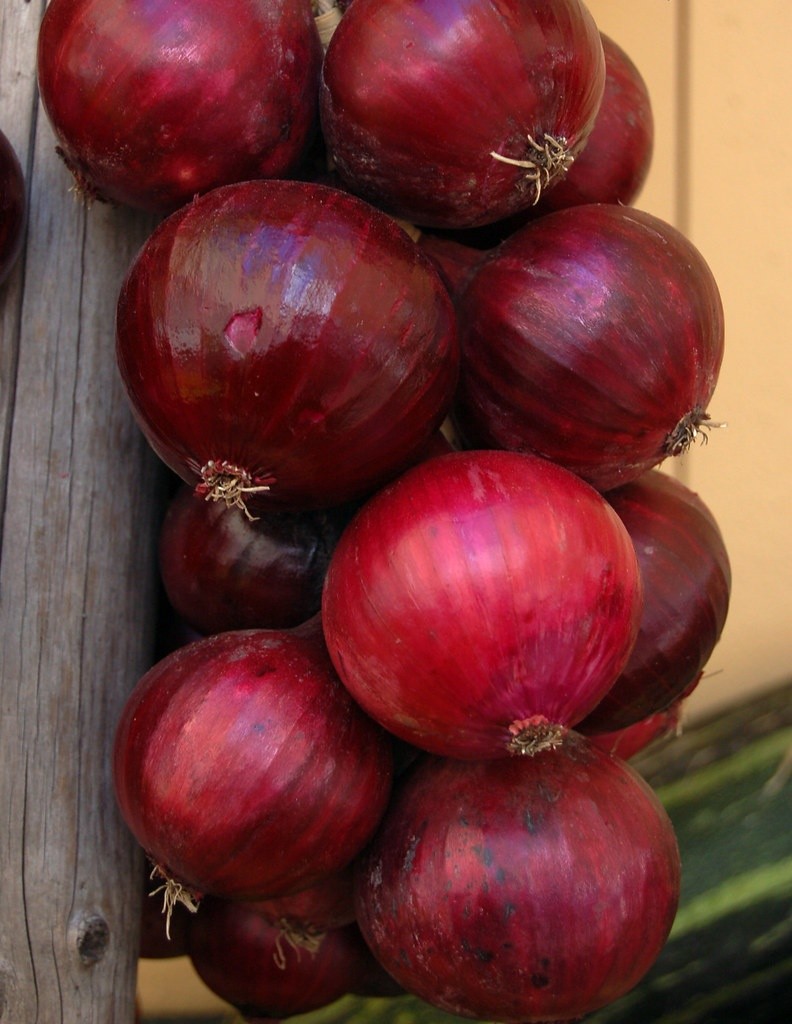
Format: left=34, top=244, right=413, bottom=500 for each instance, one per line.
left=40, top=0, right=735, bottom=1024
